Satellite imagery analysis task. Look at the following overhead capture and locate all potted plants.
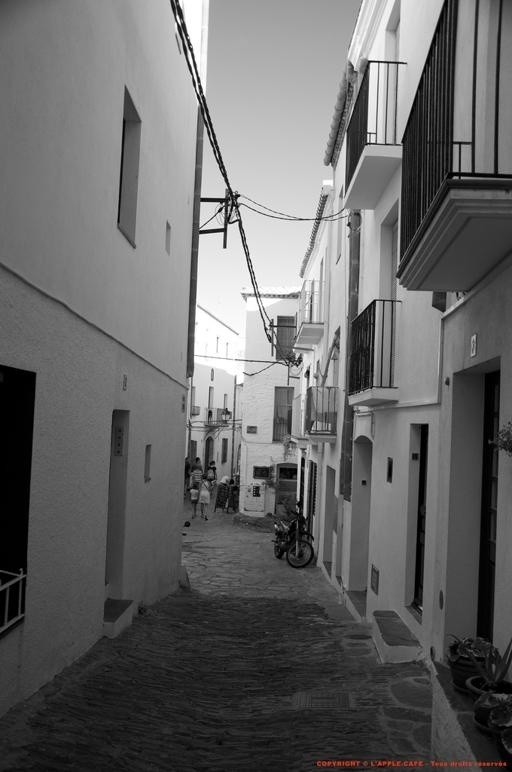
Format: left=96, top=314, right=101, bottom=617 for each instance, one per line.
left=445, top=632, right=512, bottom=772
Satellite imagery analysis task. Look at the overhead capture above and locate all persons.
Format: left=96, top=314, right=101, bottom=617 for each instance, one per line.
left=183, top=455, right=240, bottom=514
left=187, top=483, right=200, bottom=519
left=198, top=473, right=212, bottom=521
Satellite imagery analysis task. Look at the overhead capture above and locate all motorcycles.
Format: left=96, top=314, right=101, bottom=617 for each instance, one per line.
left=271, top=500, right=314, bottom=568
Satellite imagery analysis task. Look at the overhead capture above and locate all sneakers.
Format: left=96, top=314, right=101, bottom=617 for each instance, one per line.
left=192, top=513, right=197, bottom=519
left=213, top=509, right=237, bottom=514
left=200, top=513, right=208, bottom=521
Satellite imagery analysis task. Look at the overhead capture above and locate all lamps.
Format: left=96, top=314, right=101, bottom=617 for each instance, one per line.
left=221, top=408, right=230, bottom=425
